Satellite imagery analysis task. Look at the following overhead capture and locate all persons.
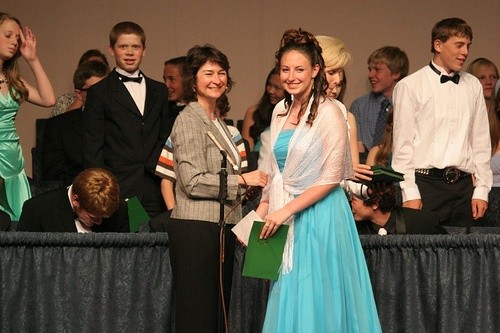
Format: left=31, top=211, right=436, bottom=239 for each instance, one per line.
left=17, top=168, right=131, bottom=234
left=236, top=28, right=383, bottom=333
left=38, top=21, right=500, bottom=232
left=391, top=17, right=494, bottom=235
left=166, top=44, right=268, bottom=333
left=0, top=12, right=57, bottom=228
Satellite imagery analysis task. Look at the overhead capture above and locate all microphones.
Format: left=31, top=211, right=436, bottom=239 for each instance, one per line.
left=207, top=131, right=241, bottom=174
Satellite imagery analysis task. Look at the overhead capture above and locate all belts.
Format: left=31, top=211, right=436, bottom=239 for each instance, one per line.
left=415, top=167, right=470, bottom=184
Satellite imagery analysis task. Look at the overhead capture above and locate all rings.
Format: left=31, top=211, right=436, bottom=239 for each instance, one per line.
left=265, top=223, right=269, bottom=227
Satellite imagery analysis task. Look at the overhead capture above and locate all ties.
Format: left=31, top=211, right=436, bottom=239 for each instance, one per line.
left=372, top=100, right=390, bottom=145
left=378, top=228, right=387, bottom=236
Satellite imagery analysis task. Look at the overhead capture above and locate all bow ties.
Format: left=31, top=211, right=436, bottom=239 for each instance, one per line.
left=429, top=61, right=459, bottom=84
left=114, top=68, right=142, bottom=83
left=72, top=211, right=78, bottom=220
